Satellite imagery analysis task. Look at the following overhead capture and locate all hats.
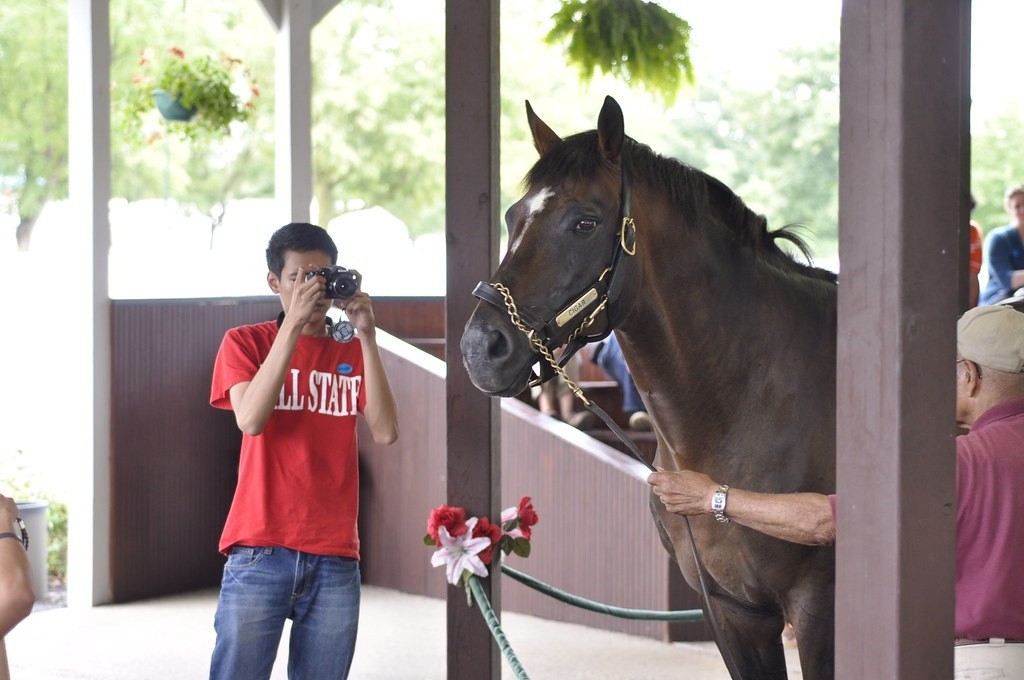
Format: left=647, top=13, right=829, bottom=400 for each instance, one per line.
left=957, top=305, right=1024, bottom=373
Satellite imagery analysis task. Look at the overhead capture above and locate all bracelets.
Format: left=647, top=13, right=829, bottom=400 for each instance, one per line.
left=0, top=532, right=23, bottom=544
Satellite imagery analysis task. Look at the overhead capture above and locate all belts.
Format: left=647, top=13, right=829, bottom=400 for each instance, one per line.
left=954, top=637, right=1024, bottom=645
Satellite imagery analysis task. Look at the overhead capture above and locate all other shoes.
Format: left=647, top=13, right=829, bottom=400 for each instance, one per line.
left=546, top=409, right=557, bottom=416
left=568, top=411, right=593, bottom=431
left=629, top=410, right=654, bottom=431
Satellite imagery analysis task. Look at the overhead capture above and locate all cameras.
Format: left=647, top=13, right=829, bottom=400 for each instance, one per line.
left=306, top=265, right=356, bottom=300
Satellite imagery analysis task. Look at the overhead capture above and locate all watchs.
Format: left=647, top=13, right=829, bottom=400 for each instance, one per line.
left=711, top=485, right=734, bottom=525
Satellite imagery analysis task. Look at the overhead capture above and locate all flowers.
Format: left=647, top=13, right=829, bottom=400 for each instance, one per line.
left=421, top=494, right=540, bottom=607
left=119, top=40, right=264, bottom=154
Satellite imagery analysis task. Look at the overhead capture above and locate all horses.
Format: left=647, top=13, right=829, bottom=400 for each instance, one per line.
left=459, top=95, right=837, bottom=680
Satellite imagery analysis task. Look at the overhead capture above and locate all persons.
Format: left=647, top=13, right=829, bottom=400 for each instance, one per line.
left=967, top=184, right=1024, bottom=310
left=538, top=330, right=653, bottom=434
left=0, top=495, right=35, bottom=680
left=207, top=223, right=399, bottom=680
left=646, top=305, right=1023, bottom=680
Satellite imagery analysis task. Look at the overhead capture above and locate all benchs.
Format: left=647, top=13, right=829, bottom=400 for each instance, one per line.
left=395, top=334, right=657, bottom=464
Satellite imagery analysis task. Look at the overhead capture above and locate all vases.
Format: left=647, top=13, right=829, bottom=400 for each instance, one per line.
left=150, top=88, right=199, bottom=123
